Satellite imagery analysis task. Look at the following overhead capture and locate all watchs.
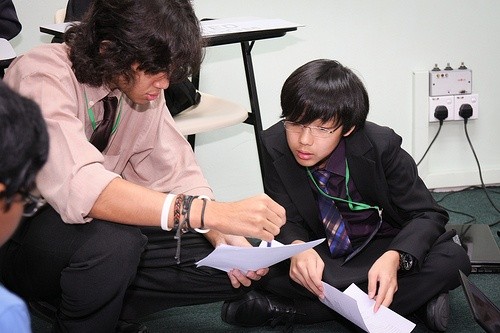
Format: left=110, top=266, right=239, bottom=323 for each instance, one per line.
left=388, top=249, right=413, bottom=272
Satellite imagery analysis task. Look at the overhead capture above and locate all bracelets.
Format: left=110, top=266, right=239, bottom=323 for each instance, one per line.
left=161, top=194, right=213, bottom=265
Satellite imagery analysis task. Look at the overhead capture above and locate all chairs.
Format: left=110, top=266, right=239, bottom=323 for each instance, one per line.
left=55, top=10, right=248, bottom=137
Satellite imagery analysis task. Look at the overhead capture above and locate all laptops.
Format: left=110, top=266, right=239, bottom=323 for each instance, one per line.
left=444, top=224, right=500, bottom=273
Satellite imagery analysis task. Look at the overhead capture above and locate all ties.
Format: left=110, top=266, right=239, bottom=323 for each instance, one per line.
left=89, top=97, right=119, bottom=152
left=313, top=169, right=353, bottom=258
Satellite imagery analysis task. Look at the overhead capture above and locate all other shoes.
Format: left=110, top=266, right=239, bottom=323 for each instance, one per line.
left=423, top=292, right=451, bottom=332
left=220, top=292, right=298, bottom=328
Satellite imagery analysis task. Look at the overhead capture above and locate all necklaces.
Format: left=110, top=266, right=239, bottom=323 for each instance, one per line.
left=85, top=87, right=123, bottom=136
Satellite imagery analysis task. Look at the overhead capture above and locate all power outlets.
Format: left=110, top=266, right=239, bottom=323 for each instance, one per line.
left=429, top=95, right=455, bottom=123
left=455, top=94, right=478, bottom=121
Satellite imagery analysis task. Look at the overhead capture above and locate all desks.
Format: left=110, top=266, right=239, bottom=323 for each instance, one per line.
left=40, top=17, right=295, bottom=195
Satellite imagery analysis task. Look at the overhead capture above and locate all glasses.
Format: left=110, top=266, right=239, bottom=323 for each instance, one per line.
left=7, top=182, right=45, bottom=218
left=279, top=116, right=343, bottom=137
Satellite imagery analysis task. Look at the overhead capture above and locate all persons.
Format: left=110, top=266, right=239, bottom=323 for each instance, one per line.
left=222, top=58, right=472, bottom=333
left=0, top=0, right=89, bottom=333
left=3, top=0, right=286, bottom=333
